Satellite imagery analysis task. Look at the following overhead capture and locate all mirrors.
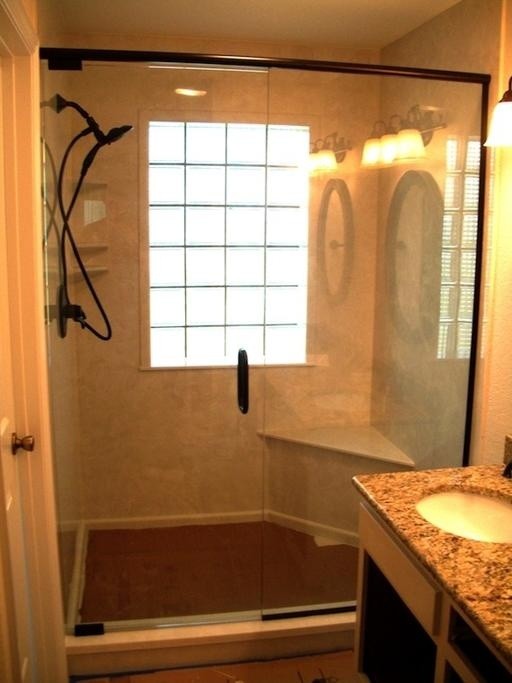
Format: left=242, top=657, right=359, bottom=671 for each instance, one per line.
left=317, top=170, right=443, bottom=346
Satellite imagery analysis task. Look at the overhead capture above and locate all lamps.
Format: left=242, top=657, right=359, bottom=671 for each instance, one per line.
left=484, top=73, right=511, bottom=152
left=308, top=106, right=448, bottom=179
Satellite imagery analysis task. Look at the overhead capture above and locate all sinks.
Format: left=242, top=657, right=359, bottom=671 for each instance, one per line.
left=415, top=484, right=512, bottom=544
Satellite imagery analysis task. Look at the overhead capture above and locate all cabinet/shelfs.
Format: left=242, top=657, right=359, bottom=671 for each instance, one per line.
left=349, top=502, right=512, bottom=683
